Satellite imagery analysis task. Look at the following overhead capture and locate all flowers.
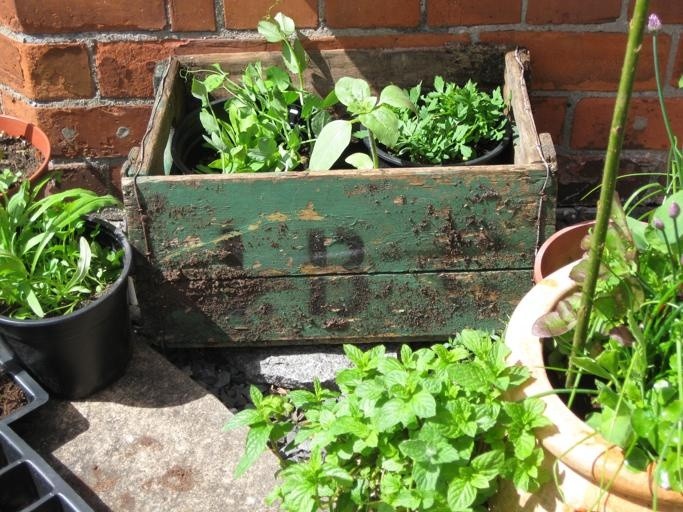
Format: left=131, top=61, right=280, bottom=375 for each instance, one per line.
left=569, top=13, right=683, bottom=484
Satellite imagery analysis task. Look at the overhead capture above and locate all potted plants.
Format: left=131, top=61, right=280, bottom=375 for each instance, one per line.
left=0, top=202, right=133, bottom=407
left=360, top=85, right=514, bottom=172
left=165, top=91, right=342, bottom=174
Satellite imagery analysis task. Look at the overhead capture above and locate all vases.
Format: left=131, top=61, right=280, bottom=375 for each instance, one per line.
left=0, top=115, right=54, bottom=192
left=532, top=213, right=650, bottom=288
left=487, top=257, right=682, bottom=512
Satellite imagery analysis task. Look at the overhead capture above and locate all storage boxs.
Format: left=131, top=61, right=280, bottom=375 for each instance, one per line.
left=123, top=40, right=564, bottom=348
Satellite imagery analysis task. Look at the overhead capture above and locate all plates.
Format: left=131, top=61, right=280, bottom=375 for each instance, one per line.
left=0, top=339, right=51, bottom=425
left=0, top=425, right=97, bottom=511
